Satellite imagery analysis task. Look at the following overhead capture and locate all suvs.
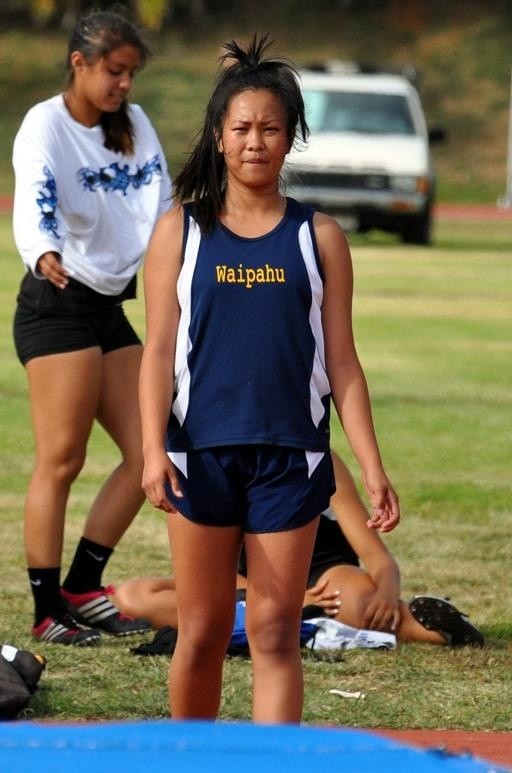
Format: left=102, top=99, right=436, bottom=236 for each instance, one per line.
left=261, top=53, right=442, bottom=254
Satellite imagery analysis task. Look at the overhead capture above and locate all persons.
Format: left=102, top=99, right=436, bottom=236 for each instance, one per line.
left=10, top=11, right=178, bottom=649
left=117, top=444, right=488, bottom=650
left=140, top=34, right=403, bottom=725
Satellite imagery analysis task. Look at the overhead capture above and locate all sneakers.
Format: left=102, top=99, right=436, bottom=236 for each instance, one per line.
left=32, top=587, right=153, bottom=645
left=408, top=596, right=484, bottom=648
